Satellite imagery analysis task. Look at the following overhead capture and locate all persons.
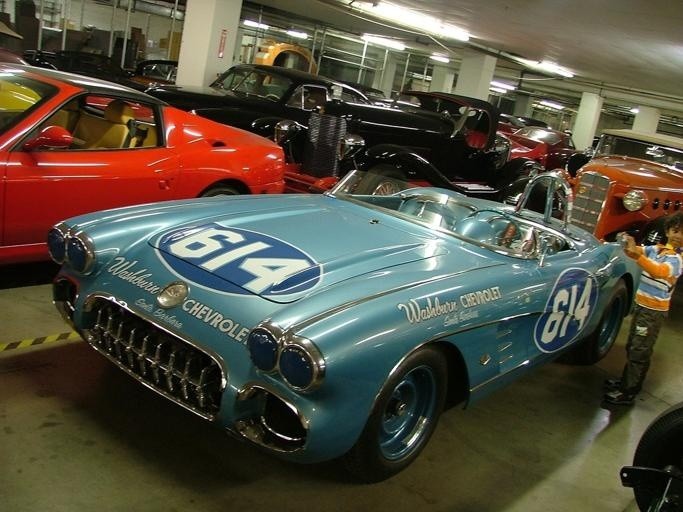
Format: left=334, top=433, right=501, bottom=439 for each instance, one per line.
left=599, top=210, right=682, bottom=407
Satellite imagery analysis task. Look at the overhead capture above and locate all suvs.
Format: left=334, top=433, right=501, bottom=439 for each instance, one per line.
left=548, top=126, right=682, bottom=243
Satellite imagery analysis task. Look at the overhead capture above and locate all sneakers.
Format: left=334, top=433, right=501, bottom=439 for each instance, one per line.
left=603, top=379, right=635, bottom=405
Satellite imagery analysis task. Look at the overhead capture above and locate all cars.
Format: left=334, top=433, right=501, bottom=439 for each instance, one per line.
left=0, top=60, right=286, bottom=267
left=143, top=63, right=376, bottom=147
left=0, top=47, right=33, bottom=66
left=134, top=60, right=179, bottom=86
left=273, top=91, right=545, bottom=205
left=338, top=80, right=421, bottom=110
left=495, top=124, right=575, bottom=174
left=22, top=49, right=148, bottom=93
left=44, top=142, right=644, bottom=474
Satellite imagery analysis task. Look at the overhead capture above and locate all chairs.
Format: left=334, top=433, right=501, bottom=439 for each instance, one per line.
left=463, top=120, right=488, bottom=149
left=47, top=99, right=80, bottom=129
left=482, top=214, right=566, bottom=252
left=71, top=99, right=138, bottom=148
left=397, top=193, right=480, bottom=231
